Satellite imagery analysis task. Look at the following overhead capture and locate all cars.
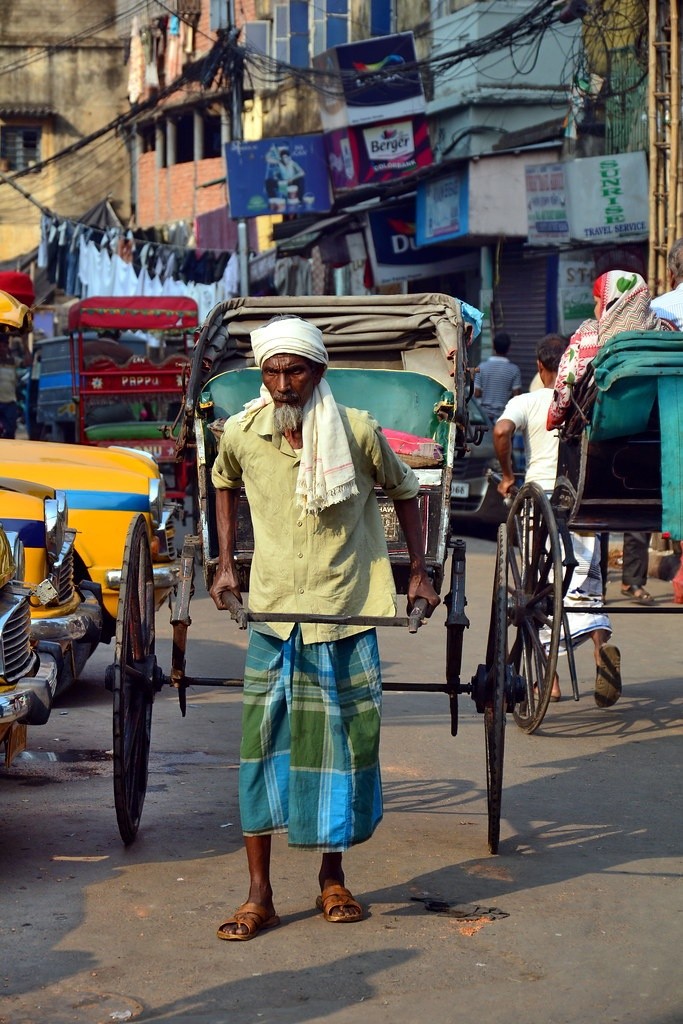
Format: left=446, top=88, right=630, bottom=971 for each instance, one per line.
left=0, top=435, right=185, bottom=775
left=451, top=418, right=527, bottom=530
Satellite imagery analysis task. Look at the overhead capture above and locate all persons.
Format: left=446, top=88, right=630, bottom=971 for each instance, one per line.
left=0, top=334, right=32, bottom=438
left=546, top=270, right=680, bottom=431
left=82, top=328, right=135, bottom=425
left=493, top=333, right=623, bottom=708
left=211, top=321, right=441, bottom=942
left=474, top=340, right=522, bottom=421
left=601, top=531, right=654, bottom=605
left=267, top=149, right=306, bottom=201
left=650, top=238, right=683, bottom=332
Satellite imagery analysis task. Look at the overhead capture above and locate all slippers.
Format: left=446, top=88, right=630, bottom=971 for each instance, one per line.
left=620, top=583, right=656, bottom=605
left=216, top=902, right=281, bottom=941
left=530, top=679, right=561, bottom=703
left=594, top=643, right=622, bottom=709
left=315, top=884, right=366, bottom=923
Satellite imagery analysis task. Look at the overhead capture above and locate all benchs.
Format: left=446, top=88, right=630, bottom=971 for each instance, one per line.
left=83, top=420, right=183, bottom=440
left=203, top=365, right=455, bottom=488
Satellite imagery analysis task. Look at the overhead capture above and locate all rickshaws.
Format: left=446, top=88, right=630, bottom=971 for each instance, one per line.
left=101, top=289, right=523, bottom=855
left=484, top=328, right=683, bottom=736
